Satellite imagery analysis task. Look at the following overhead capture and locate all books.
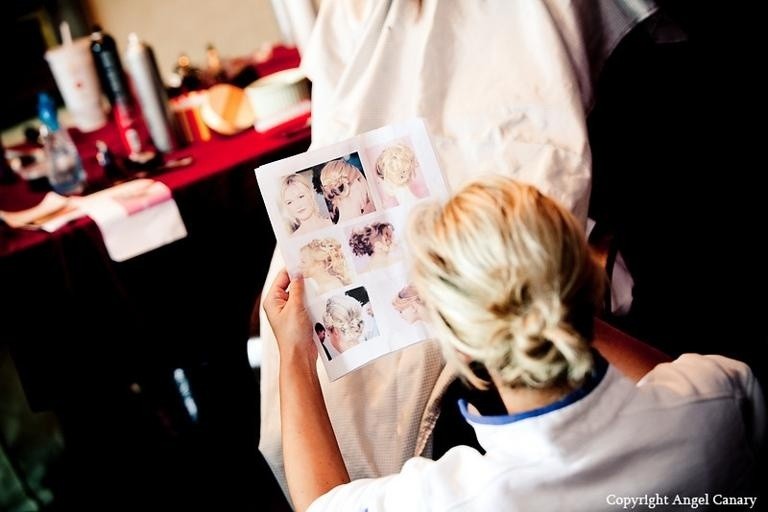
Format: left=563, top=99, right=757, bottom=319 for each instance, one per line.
left=255, top=114, right=456, bottom=383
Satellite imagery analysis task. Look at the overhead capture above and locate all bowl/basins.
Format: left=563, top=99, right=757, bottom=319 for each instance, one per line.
left=243, top=69, right=310, bottom=117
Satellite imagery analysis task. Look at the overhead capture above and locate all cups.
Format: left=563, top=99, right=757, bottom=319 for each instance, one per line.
left=42, top=38, right=108, bottom=134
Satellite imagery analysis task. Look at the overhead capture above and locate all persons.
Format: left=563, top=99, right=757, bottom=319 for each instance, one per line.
left=347, top=220, right=401, bottom=269
left=277, top=176, right=331, bottom=238
left=315, top=156, right=371, bottom=228
left=311, top=322, right=332, bottom=361
left=391, top=279, right=437, bottom=326
left=296, top=238, right=353, bottom=293
left=262, top=174, right=767, bottom=511
left=372, top=143, right=425, bottom=207
left=322, top=295, right=366, bottom=353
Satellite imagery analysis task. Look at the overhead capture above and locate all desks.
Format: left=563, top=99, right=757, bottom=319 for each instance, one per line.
left=0, top=114, right=347, bottom=451
